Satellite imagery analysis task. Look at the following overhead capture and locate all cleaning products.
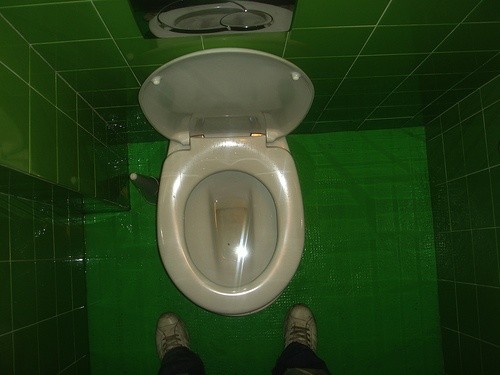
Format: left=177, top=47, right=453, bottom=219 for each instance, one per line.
left=129, top=173, right=158, bottom=205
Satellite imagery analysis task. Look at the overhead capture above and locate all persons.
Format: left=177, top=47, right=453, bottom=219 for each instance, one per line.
left=156, top=304, right=331, bottom=375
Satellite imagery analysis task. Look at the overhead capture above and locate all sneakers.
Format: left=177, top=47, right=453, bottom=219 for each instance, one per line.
left=155, top=311, right=191, bottom=361
left=282, top=303, right=319, bottom=355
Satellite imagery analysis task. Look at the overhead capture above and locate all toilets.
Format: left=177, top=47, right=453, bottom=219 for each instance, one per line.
left=137, top=47, right=316, bottom=317
left=149, top=1, right=294, bottom=39
